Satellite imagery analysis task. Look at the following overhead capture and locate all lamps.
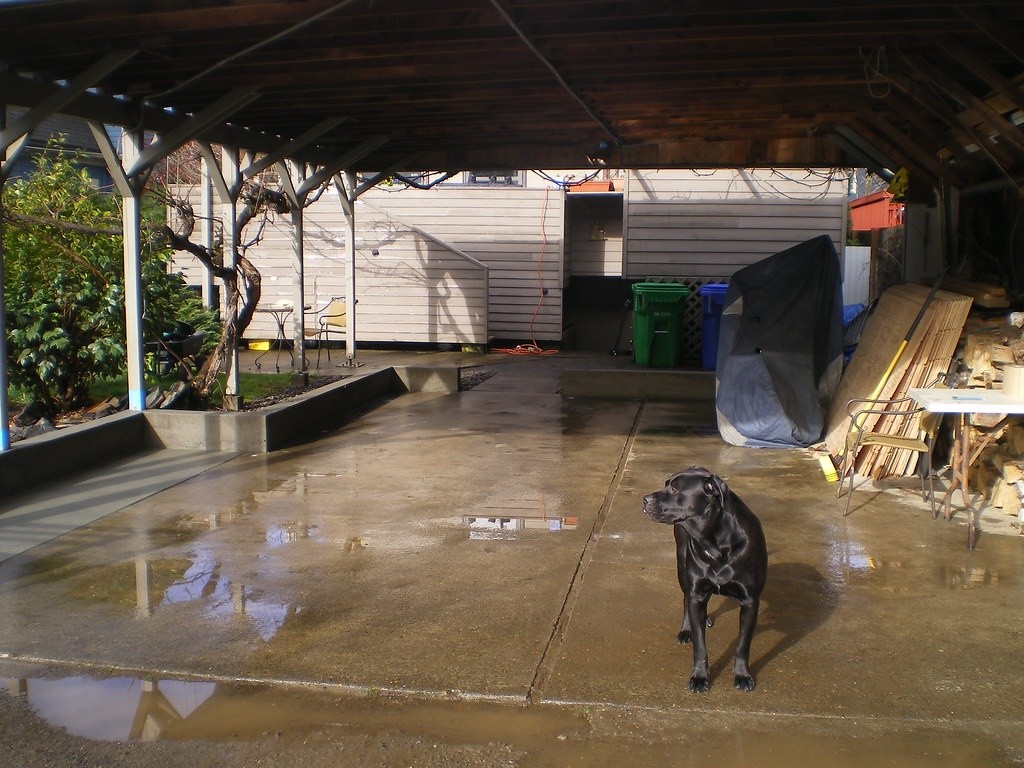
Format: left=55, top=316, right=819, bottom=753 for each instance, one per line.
left=586, top=140, right=615, bottom=167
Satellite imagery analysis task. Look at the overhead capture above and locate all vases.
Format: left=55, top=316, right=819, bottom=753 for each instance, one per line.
left=568, top=178, right=614, bottom=192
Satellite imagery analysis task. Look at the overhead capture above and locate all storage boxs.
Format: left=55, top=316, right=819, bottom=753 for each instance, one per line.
left=249, top=339, right=271, bottom=350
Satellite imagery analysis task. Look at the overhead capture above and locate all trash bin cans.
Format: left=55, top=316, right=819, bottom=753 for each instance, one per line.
left=700, top=284, right=731, bottom=368
left=632, top=281, right=690, bottom=369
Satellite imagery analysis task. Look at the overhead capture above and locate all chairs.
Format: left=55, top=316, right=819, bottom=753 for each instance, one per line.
left=305, top=296, right=358, bottom=369
left=837, top=366, right=959, bottom=519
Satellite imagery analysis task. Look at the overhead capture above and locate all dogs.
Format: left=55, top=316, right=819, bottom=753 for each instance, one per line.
left=642, top=464, right=770, bottom=695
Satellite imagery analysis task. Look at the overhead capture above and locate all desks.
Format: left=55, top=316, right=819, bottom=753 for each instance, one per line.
left=255, top=302, right=314, bottom=371
left=909, top=388, right=1024, bottom=553
